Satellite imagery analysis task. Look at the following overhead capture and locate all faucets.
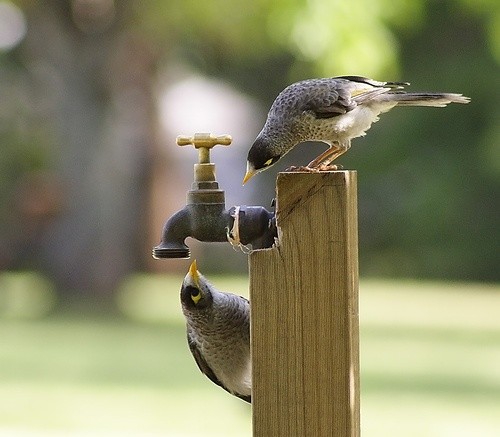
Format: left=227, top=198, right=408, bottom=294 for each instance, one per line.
left=155, top=133, right=279, bottom=258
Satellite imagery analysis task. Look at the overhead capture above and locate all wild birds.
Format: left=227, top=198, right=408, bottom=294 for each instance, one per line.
left=180, top=259, right=252, bottom=408
left=242, top=74, right=472, bottom=187
